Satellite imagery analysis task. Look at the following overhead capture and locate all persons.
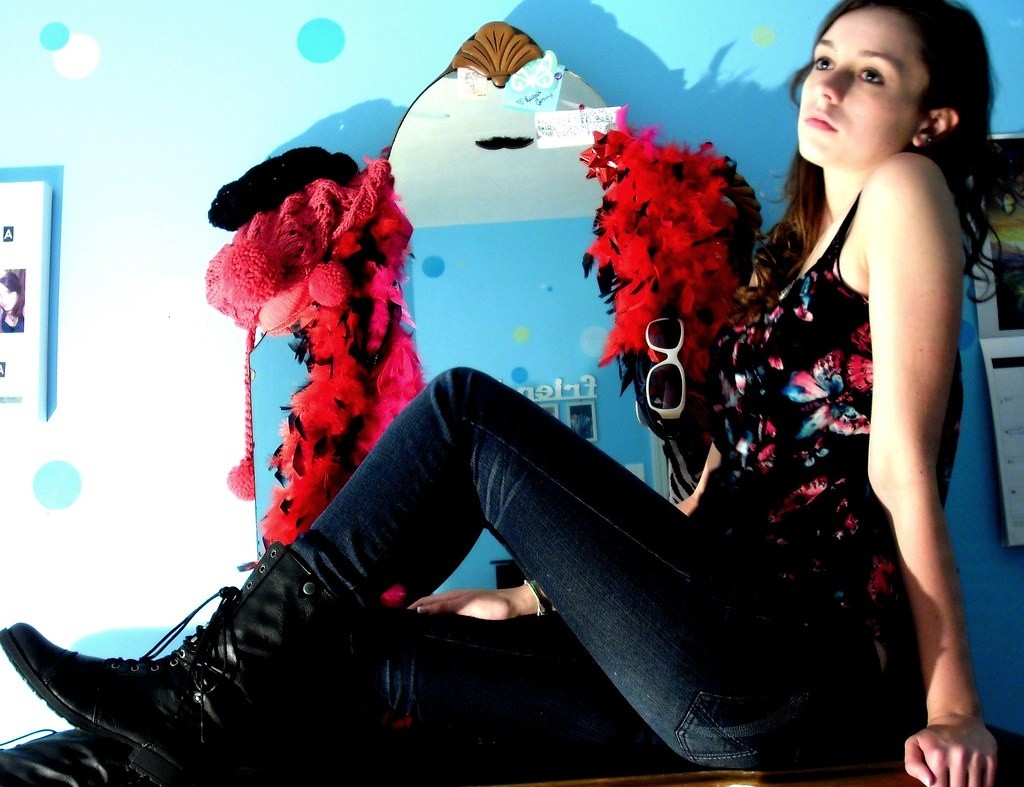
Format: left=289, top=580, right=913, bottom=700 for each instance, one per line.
left=3, top=1, right=1002, bottom=787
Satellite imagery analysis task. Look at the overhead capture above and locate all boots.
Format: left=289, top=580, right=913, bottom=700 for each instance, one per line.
left=0, top=728, right=148, bottom=787
left=0, top=539, right=353, bottom=787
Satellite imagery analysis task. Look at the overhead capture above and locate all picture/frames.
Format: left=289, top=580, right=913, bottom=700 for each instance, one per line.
left=0, top=181, right=54, bottom=426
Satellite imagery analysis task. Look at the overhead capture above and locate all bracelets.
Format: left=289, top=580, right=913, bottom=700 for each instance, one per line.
left=522, top=579, right=550, bottom=618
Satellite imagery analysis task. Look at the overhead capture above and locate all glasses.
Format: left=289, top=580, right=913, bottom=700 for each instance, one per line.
left=645, top=316, right=686, bottom=421
